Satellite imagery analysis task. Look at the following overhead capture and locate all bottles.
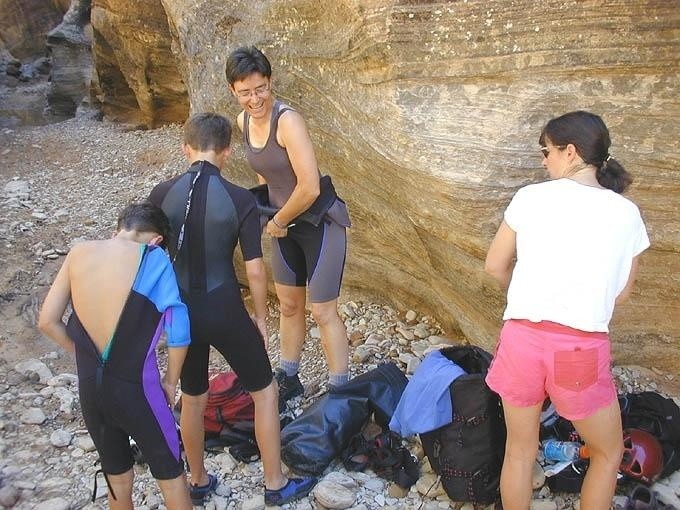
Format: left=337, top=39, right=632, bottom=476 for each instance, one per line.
left=540, top=436, right=590, bottom=462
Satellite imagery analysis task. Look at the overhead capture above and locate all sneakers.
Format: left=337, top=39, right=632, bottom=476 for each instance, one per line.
left=261, top=474, right=317, bottom=506
left=276, top=371, right=307, bottom=401
left=190, top=472, right=218, bottom=504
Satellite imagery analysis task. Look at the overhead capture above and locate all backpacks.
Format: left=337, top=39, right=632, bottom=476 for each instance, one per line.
left=171, top=370, right=286, bottom=436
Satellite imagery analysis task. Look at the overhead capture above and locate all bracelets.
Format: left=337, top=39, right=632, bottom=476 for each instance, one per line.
left=273, top=216, right=291, bottom=230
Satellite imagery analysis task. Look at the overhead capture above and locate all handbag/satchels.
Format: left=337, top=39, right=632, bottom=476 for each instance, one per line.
left=416, top=344, right=507, bottom=503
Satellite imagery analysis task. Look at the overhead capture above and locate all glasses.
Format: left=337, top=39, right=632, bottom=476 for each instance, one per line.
left=540, top=144, right=567, bottom=158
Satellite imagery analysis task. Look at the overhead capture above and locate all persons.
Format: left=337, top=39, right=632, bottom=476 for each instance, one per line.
left=223, top=40, right=353, bottom=408
left=38, top=199, right=198, bottom=510
left=139, top=107, right=316, bottom=508
left=482, top=109, right=654, bottom=510
